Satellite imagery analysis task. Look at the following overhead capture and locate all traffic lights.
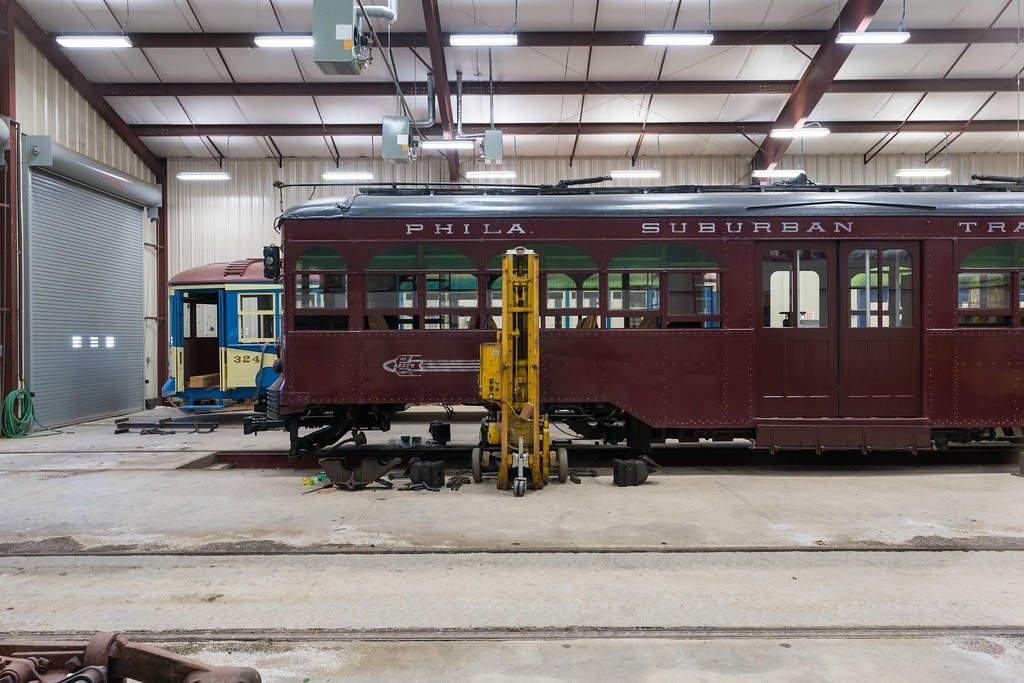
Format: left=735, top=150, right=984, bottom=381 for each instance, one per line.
left=264, top=247, right=280, bottom=280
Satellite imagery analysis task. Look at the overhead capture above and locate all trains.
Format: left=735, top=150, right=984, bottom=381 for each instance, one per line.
left=162, top=258, right=404, bottom=416
left=262, top=169, right=1024, bottom=454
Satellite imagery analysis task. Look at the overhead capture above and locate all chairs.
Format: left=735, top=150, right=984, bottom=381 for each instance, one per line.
left=367, top=314, right=660, bottom=330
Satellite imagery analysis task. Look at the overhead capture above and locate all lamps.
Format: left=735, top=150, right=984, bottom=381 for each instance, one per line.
left=57, top=0, right=953, bottom=182
